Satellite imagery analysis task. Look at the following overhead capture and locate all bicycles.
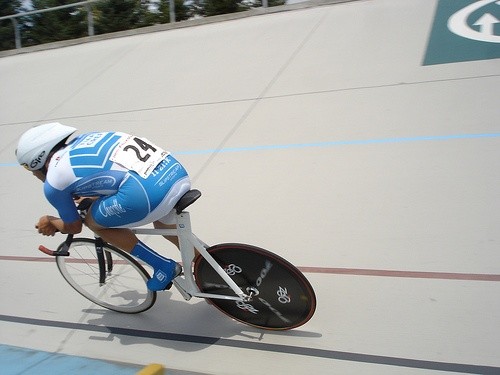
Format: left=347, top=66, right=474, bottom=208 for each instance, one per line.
left=38, top=188, right=317, bottom=331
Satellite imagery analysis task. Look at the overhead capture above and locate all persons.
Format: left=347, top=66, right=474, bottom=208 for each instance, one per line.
left=14, top=122, right=205, bottom=292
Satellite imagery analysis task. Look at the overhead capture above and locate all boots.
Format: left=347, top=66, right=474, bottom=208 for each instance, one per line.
left=131, top=240, right=182, bottom=292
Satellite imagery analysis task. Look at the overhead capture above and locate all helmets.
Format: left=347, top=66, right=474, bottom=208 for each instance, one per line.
left=14, top=123, right=77, bottom=171
